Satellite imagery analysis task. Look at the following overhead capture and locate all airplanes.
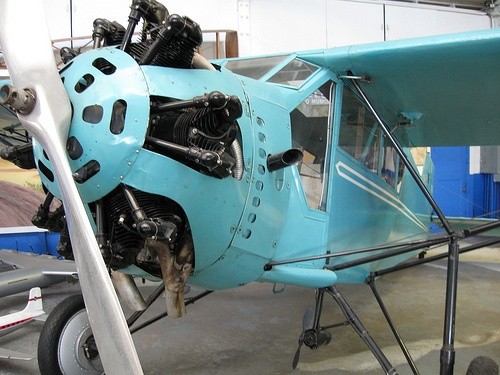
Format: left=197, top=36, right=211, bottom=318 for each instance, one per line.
left=0, top=0, right=499, bottom=375
